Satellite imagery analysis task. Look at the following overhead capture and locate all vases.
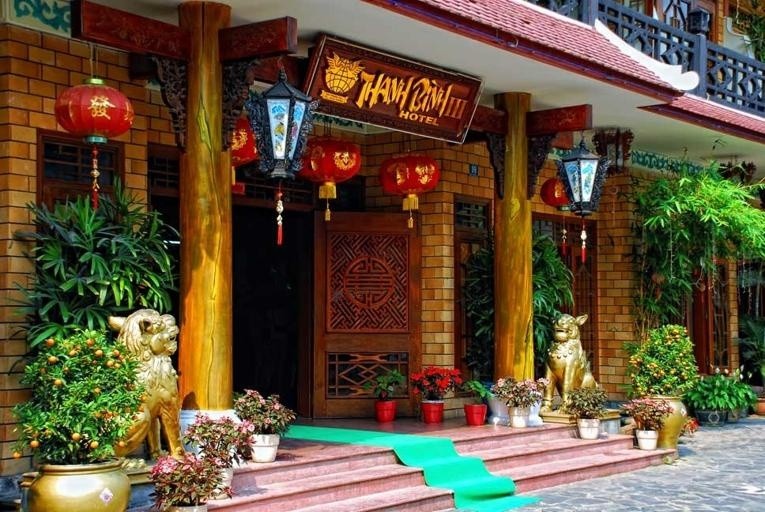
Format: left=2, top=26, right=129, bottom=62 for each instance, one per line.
left=164, top=494, right=210, bottom=509
left=205, top=462, right=238, bottom=501
left=638, top=428, right=658, bottom=450
left=420, top=398, right=445, bottom=425
left=697, top=406, right=743, bottom=426
left=508, top=405, right=528, bottom=430
left=249, top=430, right=280, bottom=463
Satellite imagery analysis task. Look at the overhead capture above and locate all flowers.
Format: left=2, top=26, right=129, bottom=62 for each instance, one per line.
left=150, top=451, right=237, bottom=507
left=620, top=397, right=672, bottom=429
left=492, top=376, right=551, bottom=405
left=234, top=390, right=299, bottom=432
left=687, top=367, right=760, bottom=413
left=411, top=365, right=463, bottom=398
left=182, top=417, right=253, bottom=465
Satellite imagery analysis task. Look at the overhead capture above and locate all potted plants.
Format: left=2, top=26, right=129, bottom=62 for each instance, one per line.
left=625, top=326, right=700, bottom=450
left=363, top=367, right=405, bottom=423
left=564, top=388, right=609, bottom=440
left=461, top=381, right=492, bottom=428
left=11, top=329, right=148, bottom=511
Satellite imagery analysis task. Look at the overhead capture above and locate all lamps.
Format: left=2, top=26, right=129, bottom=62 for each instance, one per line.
left=247, top=58, right=323, bottom=179
left=557, top=139, right=608, bottom=218
left=597, top=128, right=633, bottom=174
